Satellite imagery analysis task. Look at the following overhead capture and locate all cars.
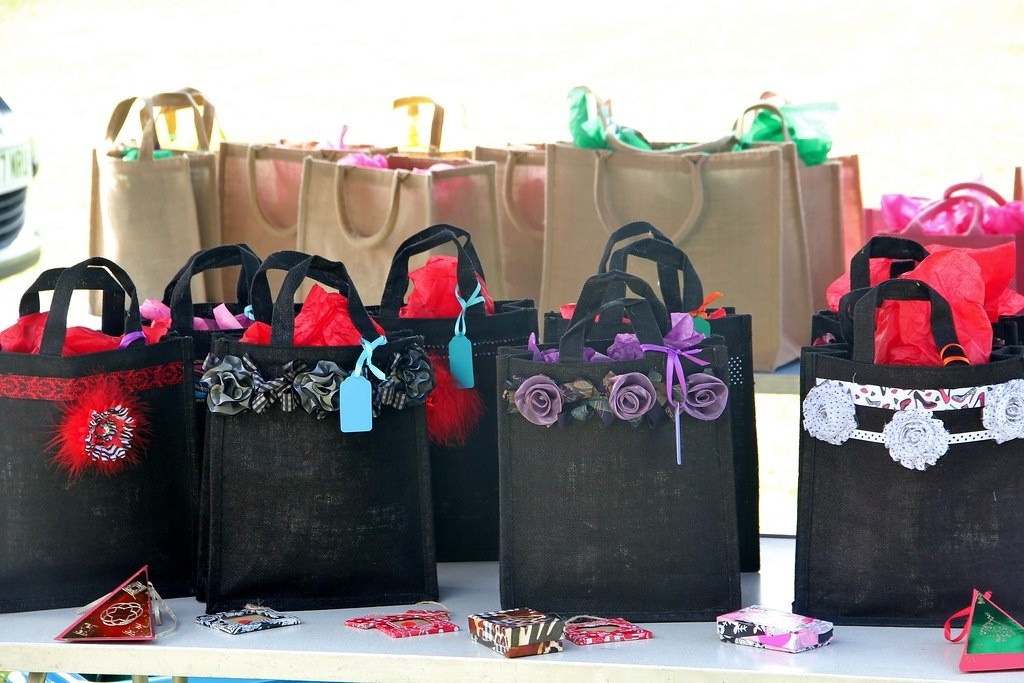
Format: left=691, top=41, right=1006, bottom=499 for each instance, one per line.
left=0, top=97, right=38, bottom=249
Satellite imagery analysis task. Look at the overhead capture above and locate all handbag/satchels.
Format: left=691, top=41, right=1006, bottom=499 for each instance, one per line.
left=0, top=85, right=1024, bottom=622
